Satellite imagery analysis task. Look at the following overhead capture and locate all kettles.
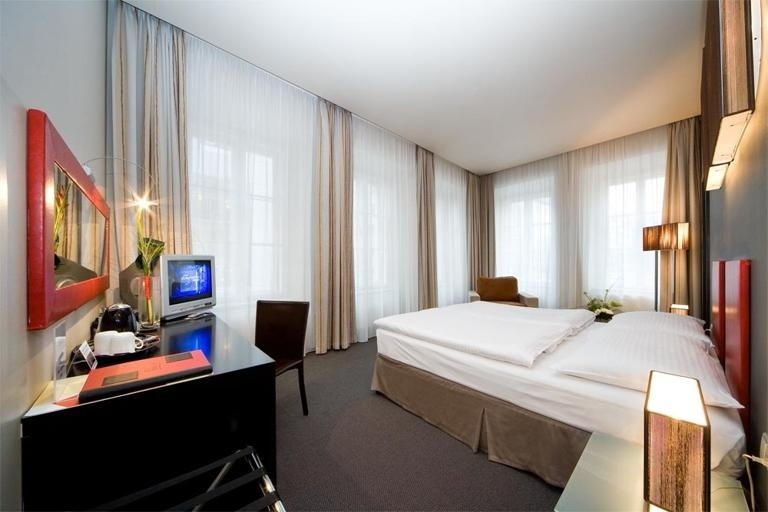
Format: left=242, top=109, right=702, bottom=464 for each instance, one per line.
left=100, top=303, right=140, bottom=334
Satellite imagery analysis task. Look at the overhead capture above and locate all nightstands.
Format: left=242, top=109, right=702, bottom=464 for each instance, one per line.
left=555, top=430, right=749, bottom=511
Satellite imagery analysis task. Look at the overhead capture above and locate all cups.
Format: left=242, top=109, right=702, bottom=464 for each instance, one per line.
left=93, top=330, right=118, bottom=356
left=112, top=331, right=144, bottom=354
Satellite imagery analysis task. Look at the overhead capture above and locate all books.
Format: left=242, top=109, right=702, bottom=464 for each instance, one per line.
left=77, top=349, right=214, bottom=403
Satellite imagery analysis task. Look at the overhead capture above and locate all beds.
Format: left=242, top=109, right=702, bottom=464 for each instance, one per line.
left=372, top=298, right=747, bottom=489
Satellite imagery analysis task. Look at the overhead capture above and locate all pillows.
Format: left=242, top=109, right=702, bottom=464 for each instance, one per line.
left=608, top=311, right=714, bottom=345
left=563, top=325, right=745, bottom=410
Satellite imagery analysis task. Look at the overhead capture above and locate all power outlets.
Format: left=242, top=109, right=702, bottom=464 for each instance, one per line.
left=758, top=433, right=768, bottom=469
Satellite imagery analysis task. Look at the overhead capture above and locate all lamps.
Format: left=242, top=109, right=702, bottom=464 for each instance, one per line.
left=642, top=222, right=689, bottom=312
left=671, top=303, right=689, bottom=316
left=643, top=369, right=712, bottom=511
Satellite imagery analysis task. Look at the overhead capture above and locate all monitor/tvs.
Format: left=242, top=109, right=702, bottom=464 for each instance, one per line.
left=121, top=256, right=216, bottom=321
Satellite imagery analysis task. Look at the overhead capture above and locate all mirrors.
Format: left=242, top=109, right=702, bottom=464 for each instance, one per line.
left=25, top=108, right=111, bottom=331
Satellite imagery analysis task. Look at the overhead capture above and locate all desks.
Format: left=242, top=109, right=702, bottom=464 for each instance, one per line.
left=21, top=312, right=277, bottom=511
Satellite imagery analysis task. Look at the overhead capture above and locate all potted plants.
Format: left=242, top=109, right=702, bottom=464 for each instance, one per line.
left=583, top=289, right=622, bottom=320
left=138, top=219, right=161, bottom=330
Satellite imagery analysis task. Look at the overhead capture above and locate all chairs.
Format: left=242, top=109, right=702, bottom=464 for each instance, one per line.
left=255, top=297, right=310, bottom=418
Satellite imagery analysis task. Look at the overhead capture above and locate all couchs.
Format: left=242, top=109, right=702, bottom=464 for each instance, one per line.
left=469, top=273, right=540, bottom=307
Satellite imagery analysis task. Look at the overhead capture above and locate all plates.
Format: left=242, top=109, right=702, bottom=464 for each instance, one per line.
left=72, top=335, right=161, bottom=354
